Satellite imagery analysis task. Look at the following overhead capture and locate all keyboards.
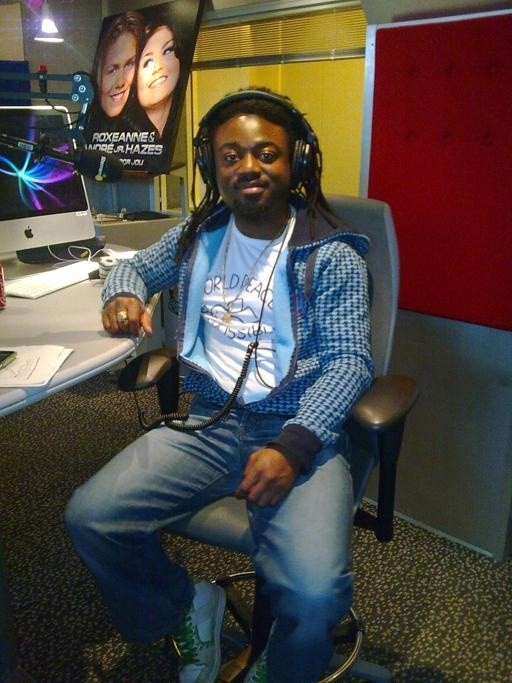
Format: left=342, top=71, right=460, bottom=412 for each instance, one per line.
left=4, top=260, right=99, bottom=300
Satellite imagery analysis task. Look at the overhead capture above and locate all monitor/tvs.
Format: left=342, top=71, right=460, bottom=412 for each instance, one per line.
left=0, top=105, right=96, bottom=253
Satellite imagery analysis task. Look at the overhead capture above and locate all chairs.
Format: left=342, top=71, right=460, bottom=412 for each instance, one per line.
left=117, top=192, right=424, bottom=683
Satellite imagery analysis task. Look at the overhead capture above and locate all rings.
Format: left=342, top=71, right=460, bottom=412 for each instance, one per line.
left=115, top=311, right=129, bottom=323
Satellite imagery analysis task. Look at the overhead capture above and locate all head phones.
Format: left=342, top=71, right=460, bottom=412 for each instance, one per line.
left=191, top=89, right=319, bottom=188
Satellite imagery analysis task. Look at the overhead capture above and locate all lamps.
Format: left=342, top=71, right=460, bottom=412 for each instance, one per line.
left=34, top=1, right=65, bottom=43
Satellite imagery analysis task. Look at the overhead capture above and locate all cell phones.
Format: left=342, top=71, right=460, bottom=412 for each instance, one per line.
left=0, top=350, right=17, bottom=369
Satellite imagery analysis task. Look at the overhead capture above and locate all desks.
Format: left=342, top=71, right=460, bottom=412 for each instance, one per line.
left=0, top=240, right=163, bottom=420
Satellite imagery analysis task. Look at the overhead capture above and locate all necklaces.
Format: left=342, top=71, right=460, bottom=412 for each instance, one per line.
left=221, top=214, right=290, bottom=324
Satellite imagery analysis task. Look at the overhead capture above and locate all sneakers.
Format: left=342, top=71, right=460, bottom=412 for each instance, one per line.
left=171, top=580, right=227, bottom=683
left=244, top=619, right=279, bottom=683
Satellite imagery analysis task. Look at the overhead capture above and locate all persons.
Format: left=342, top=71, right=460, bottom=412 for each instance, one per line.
left=110, top=14, right=190, bottom=175
left=80, top=8, right=148, bottom=168
left=61, top=83, right=377, bottom=683
